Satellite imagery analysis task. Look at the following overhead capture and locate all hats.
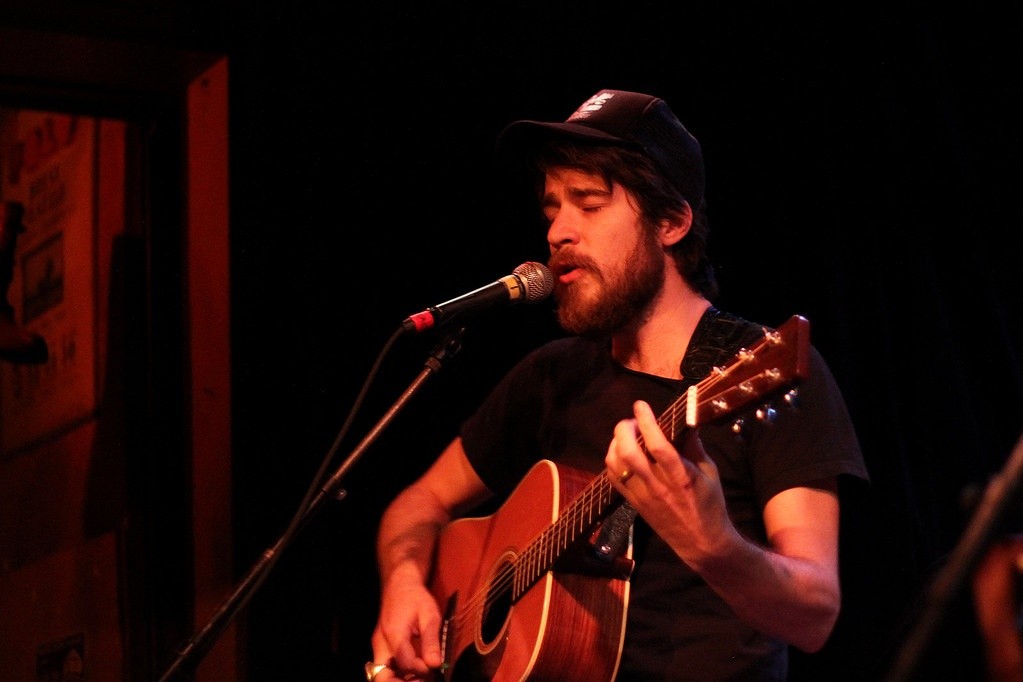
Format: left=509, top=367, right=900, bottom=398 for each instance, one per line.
left=496, top=89, right=706, bottom=211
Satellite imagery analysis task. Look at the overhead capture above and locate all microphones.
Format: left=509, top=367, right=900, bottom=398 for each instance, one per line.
left=403, top=262, right=554, bottom=334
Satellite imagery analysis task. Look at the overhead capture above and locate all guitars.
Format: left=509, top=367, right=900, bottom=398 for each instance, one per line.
left=407, top=314, right=809, bottom=682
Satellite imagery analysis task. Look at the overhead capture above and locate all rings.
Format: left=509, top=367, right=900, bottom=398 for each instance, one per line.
left=365, top=663, right=391, bottom=681
left=617, top=467, right=633, bottom=481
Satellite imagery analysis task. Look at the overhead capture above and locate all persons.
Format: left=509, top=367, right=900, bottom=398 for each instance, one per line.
left=371, top=89, right=873, bottom=682
